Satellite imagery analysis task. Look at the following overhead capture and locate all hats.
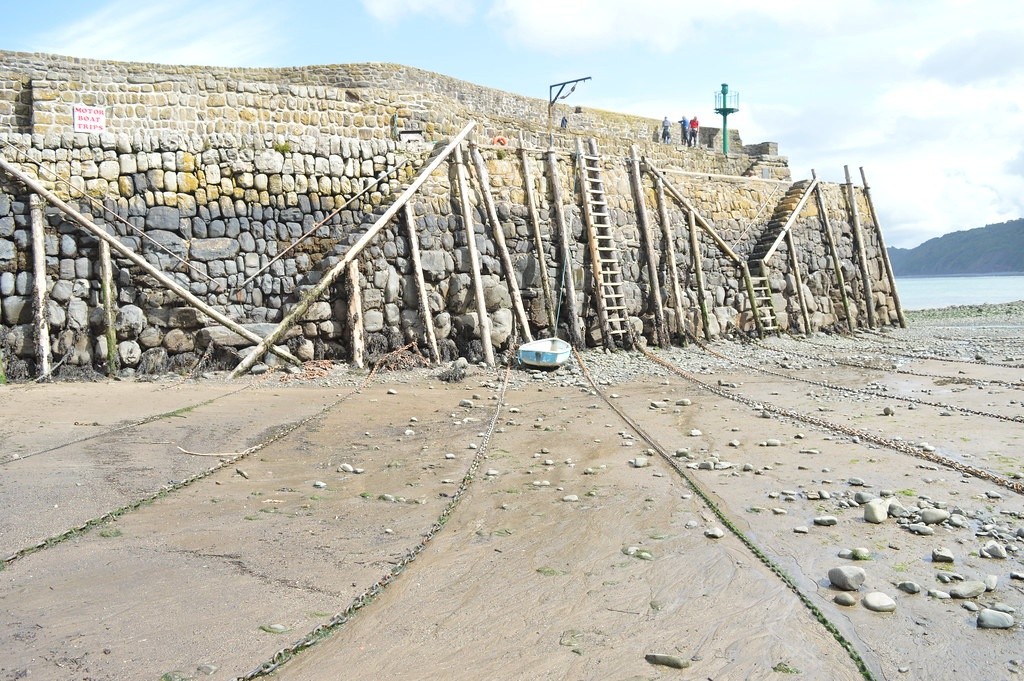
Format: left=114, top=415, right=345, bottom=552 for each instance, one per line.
left=665, top=115, right=668, bottom=118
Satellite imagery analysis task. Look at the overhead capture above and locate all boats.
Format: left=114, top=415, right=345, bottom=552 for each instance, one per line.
left=517, top=336, right=572, bottom=367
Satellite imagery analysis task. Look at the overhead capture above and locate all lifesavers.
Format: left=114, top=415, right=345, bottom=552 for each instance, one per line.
left=493, top=135, right=507, bottom=146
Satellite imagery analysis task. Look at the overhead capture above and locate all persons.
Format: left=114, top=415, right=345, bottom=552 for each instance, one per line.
left=662, top=117, right=671, bottom=143
left=678, top=116, right=699, bottom=147
